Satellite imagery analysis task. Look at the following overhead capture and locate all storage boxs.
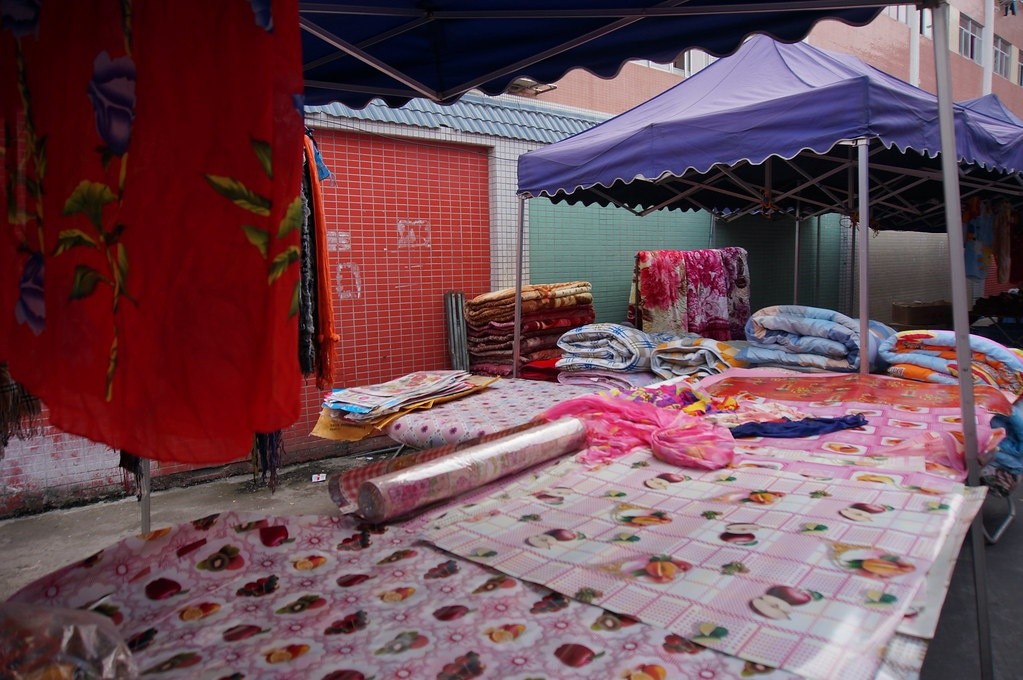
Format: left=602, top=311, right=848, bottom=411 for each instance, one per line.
left=891, top=300, right=951, bottom=324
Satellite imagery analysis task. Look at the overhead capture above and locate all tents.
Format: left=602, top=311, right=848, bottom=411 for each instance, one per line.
left=513, top=34, right=1023, bottom=375
left=300, top=0, right=994, bottom=680
